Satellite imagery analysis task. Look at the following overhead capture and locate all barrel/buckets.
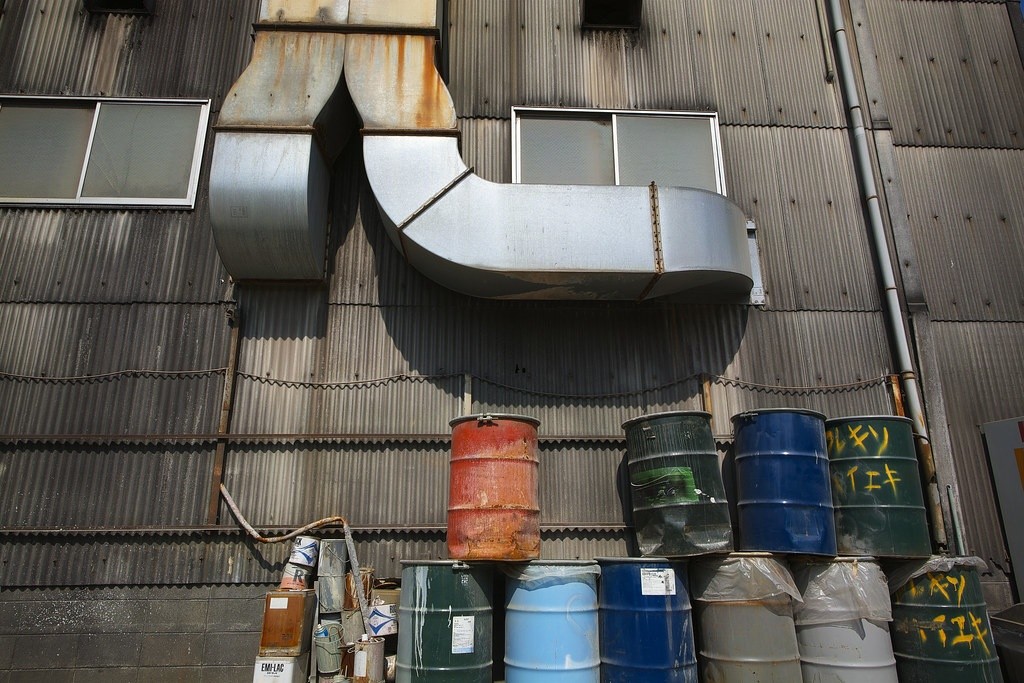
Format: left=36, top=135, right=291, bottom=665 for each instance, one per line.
left=249, top=408, right=1004, bottom=682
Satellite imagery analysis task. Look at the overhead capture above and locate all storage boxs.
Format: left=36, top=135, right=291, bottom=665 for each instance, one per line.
left=253, top=650, right=310, bottom=683
left=258, top=591, right=315, bottom=657
